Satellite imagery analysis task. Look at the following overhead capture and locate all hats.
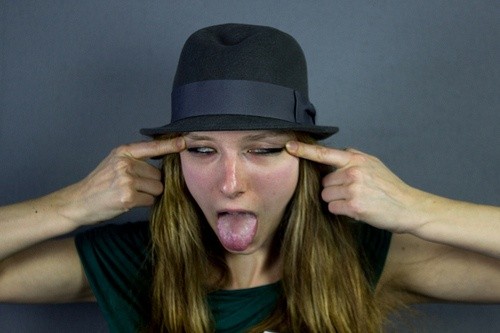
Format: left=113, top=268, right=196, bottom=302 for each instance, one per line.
left=138, top=21, right=339, bottom=140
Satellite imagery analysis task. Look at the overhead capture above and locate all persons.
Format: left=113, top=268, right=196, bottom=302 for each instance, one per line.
left=0, top=21, right=500, bottom=332
